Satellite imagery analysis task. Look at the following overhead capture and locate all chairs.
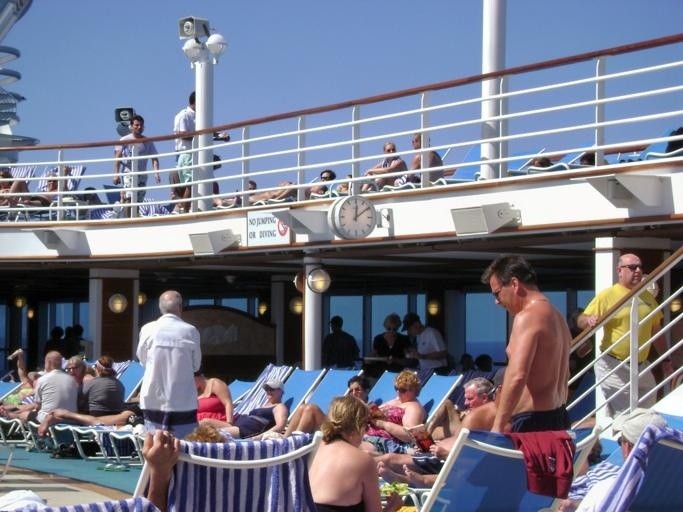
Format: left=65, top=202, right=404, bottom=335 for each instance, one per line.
left=104, top=183, right=124, bottom=203
left=0, top=165, right=86, bottom=220
left=434, top=143, right=478, bottom=178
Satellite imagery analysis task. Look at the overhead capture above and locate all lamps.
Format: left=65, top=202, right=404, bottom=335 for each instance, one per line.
left=187, top=226, right=242, bottom=257
left=448, top=201, right=524, bottom=239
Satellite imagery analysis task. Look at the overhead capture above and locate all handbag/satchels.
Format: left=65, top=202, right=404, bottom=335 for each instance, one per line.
left=53, top=441, right=101, bottom=459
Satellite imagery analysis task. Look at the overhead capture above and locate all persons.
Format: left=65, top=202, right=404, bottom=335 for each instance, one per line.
left=1, top=312, right=683, bottom=511
left=135, top=289, right=201, bottom=438
left=230, top=133, right=598, bottom=213
left=1, top=91, right=229, bottom=224
left=577, top=253, right=674, bottom=416
left=479, top=253, right=574, bottom=501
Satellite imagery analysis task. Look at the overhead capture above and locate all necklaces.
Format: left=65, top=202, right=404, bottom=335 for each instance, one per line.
left=521, top=296, right=550, bottom=307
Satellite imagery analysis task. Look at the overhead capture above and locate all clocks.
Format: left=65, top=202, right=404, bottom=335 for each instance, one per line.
left=328, top=193, right=381, bottom=240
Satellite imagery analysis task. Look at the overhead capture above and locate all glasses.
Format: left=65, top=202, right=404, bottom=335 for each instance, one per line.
left=321, top=177, right=327, bottom=179
left=388, top=327, right=395, bottom=330
left=265, top=387, right=275, bottom=391
left=617, top=436, right=622, bottom=445
left=395, top=386, right=408, bottom=392
left=388, top=149, right=394, bottom=151
left=621, top=265, right=642, bottom=269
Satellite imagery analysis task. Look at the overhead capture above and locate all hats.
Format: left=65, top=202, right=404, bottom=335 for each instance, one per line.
left=615, top=408, right=667, bottom=444
left=402, top=313, right=419, bottom=332
left=28, top=371, right=44, bottom=380
left=263, top=380, right=283, bottom=390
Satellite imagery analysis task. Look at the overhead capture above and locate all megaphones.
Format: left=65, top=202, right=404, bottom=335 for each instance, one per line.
left=176, top=16, right=210, bottom=40
left=114, top=107, right=136, bottom=123
